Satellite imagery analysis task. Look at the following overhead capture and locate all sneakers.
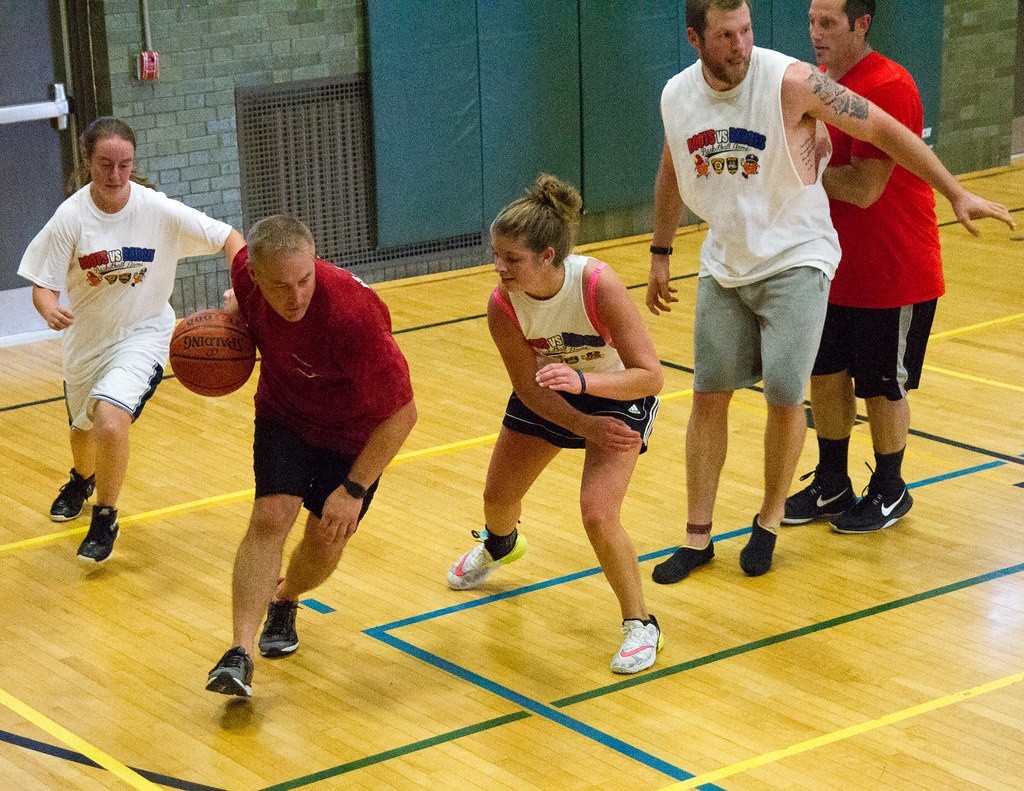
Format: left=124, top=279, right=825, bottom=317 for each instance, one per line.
left=826, top=461, right=914, bottom=536
left=48, top=466, right=96, bottom=523
left=445, top=528, right=529, bottom=592
left=257, top=576, right=304, bottom=659
left=778, top=463, right=859, bottom=526
left=608, top=611, right=666, bottom=675
left=75, top=503, right=121, bottom=567
left=204, top=645, right=254, bottom=698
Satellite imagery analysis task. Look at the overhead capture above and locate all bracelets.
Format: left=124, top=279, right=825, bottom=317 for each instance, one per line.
left=647, top=239, right=671, bottom=256
left=575, top=369, right=586, bottom=395
left=340, top=476, right=370, bottom=499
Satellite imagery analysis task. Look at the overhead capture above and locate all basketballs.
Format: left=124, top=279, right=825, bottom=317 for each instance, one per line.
left=170, top=309, right=257, bottom=396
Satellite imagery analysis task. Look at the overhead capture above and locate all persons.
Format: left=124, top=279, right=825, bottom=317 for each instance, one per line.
left=445, top=175, right=663, bottom=676
left=205, top=213, right=417, bottom=698
left=647, top=0, right=1016, bottom=585
left=16, top=117, right=247, bottom=565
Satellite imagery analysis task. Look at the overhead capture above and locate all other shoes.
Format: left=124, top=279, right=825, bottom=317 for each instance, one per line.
left=650, top=535, right=716, bottom=586
left=738, top=509, right=778, bottom=578
